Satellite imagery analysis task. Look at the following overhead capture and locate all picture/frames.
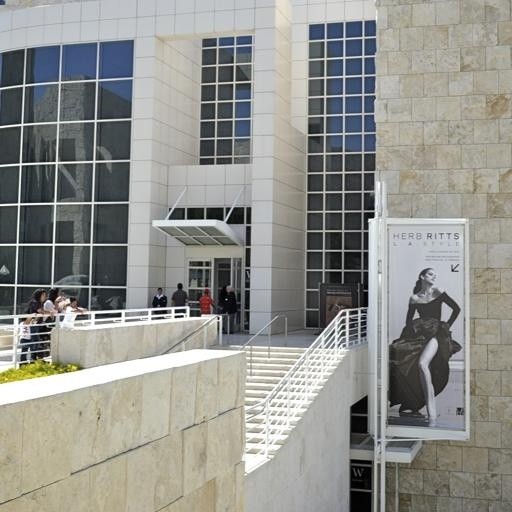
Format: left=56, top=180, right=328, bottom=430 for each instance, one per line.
left=370, top=217, right=470, bottom=442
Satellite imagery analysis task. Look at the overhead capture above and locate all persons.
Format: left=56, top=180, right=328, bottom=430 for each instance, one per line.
left=172, top=283, right=188, bottom=319
left=389, top=265, right=463, bottom=423
left=200, top=288, right=218, bottom=315
left=19, top=288, right=84, bottom=366
left=152, top=287, right=167, bottom=319
left=219, top=284, right=238, bottom=334
